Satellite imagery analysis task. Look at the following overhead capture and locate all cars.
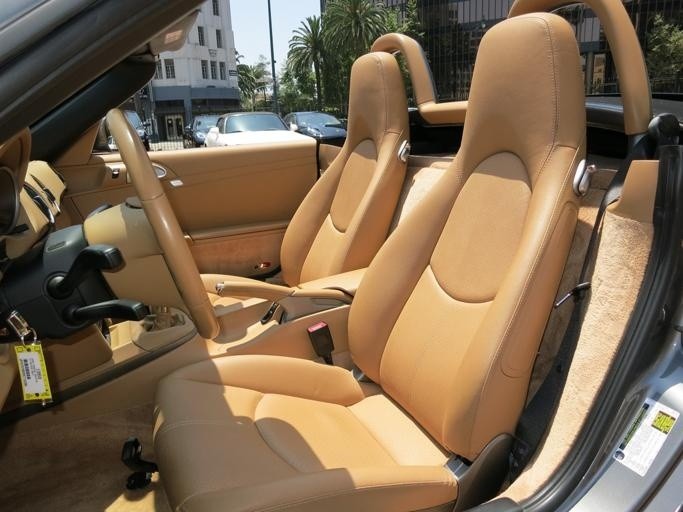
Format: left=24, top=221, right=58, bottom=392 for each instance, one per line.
left=101, top=111, right=149, bottom=155
left=184, top=112, right=348, bottom=148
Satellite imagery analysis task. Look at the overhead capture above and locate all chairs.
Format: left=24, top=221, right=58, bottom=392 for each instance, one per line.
left=151, top=11, right=587, bottom=509
left=127, top=52, right=410, bottom=401
